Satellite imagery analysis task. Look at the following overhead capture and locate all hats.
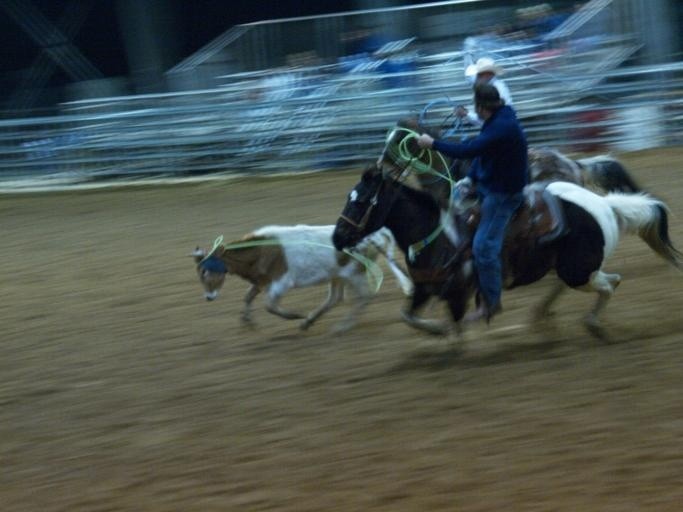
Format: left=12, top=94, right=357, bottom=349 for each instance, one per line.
left=477, top=86, right=505, bottom=111
left=466, top=57, right=503, bottom=76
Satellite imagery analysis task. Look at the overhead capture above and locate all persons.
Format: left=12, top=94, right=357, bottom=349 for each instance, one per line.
left=416, top=82, right=528, bottom=325
left=453, top=54, right=515, bottom=130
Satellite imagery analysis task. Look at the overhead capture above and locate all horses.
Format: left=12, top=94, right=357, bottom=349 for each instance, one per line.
left=376, top=115, right=641, bottom=199
left=332, top=160, right=682, bottom=356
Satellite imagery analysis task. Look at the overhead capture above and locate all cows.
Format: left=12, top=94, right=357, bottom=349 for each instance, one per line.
left=187, top=223, right=416, bottom=332
